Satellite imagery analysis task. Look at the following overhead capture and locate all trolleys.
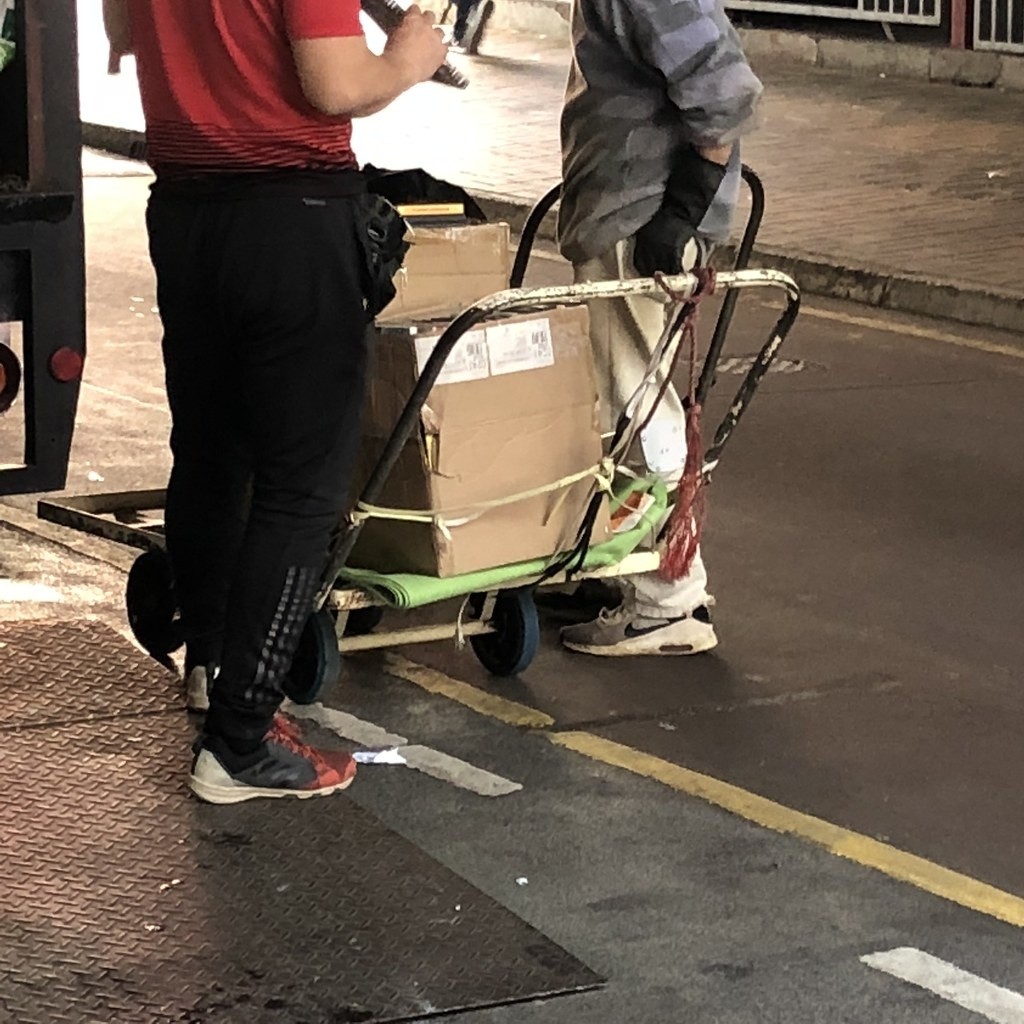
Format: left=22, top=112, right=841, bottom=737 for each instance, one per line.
left=38, top=267, right=803, bottom=706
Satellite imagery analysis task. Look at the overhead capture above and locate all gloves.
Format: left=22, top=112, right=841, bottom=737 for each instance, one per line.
left=633, top=143, right=728, bottom=279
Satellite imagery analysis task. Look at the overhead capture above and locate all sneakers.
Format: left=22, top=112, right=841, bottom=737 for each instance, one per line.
left=558, top=594, right=719, bottom=657
left=185, top=712, right=358, bottom=804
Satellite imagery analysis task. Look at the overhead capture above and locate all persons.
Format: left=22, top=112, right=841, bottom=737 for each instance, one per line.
left=556, top=0, right=764, bottom=655
left=102, top=0, right=449, bottom=804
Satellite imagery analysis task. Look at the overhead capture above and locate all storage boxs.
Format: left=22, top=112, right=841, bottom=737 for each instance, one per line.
left=337, top=217, right=612, bottom=578
